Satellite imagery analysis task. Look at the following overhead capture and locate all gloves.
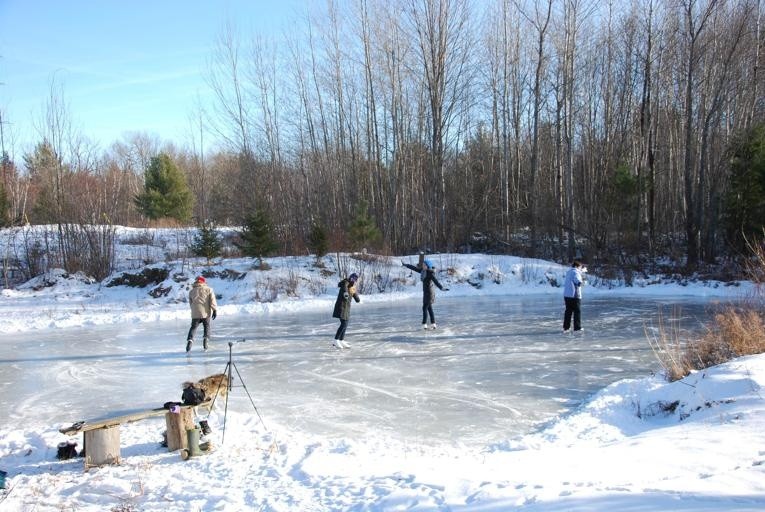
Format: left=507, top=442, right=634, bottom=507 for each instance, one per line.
left=212, top=310, right=217, bottom=320
left=441, top=288, right=450, bottom=291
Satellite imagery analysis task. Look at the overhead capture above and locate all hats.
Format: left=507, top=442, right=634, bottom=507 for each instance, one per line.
left=424, top=260, right=432, bottom=269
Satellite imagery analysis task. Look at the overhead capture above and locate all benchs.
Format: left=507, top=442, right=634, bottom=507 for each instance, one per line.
left=59, top=396, right=212, bottom=470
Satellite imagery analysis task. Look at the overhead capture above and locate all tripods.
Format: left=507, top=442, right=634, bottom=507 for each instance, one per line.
left=198, top=345, right=281, bottom=453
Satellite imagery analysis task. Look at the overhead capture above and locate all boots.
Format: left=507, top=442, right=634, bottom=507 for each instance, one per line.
left=340, top=340, right=351, bottom=349
left=333, top=340, right=343, bottom=347
left=564, top=328, right=584, bottom=334
left=422, top=323, right=436, bottom=329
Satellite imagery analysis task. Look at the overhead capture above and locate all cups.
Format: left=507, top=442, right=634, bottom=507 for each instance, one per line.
left=186, top=428, right=204, bottom=455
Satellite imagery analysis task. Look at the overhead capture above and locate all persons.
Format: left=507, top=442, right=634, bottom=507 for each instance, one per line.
left=401, top=259, right=449, bottom=329
left=562, top=261, right=584, bottom=333
left=331, top=272, right=360, bottom=349
left=186, top=276, right=217, bottom=352
